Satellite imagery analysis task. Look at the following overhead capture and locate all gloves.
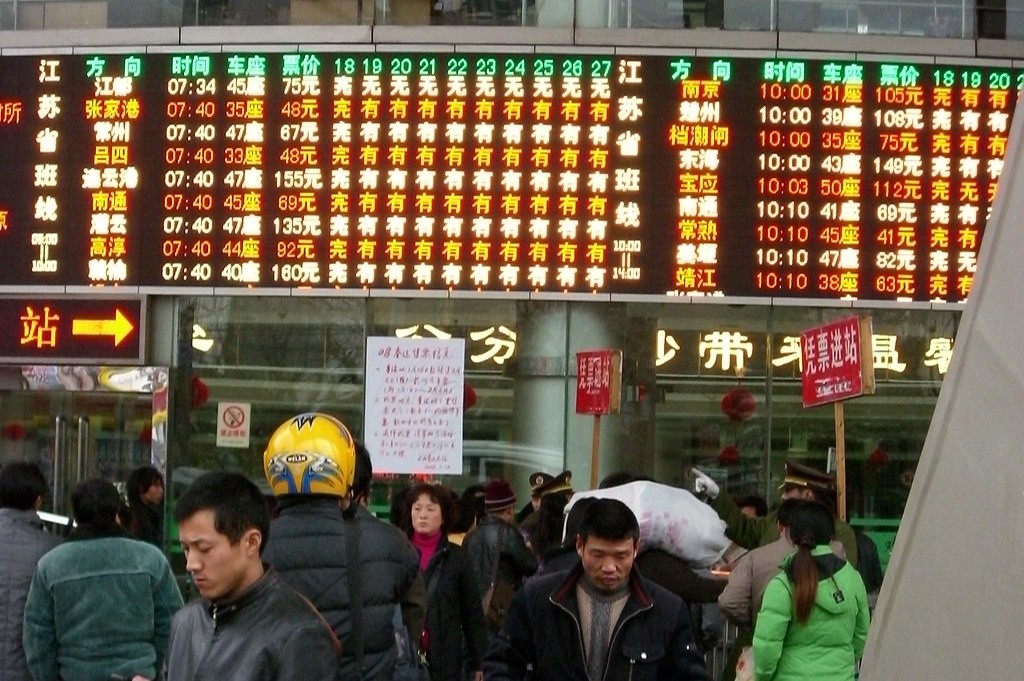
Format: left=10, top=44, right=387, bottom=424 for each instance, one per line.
left=692, top=468, right=719, bottom=500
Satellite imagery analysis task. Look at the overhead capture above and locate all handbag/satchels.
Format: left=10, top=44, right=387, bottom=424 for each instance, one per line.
left=480, top=579, right=517, bottom=628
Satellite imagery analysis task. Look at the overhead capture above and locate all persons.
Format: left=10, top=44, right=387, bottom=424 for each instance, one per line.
left=0, top=411, right=879, bottom=681
left=124, top=468, right=349, bottom=681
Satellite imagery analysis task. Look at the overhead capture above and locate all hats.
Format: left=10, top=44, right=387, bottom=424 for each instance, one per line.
left=528, top=471, right=555, bottom=496
left=533, top=471, right=574, bottom=499
left=484, top=480, right=515, bottom=511
left=777, top=457, right=832, bottom=493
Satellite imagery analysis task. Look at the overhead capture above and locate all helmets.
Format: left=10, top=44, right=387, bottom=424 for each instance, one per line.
left=263, top=413, right=355, bottom=496
